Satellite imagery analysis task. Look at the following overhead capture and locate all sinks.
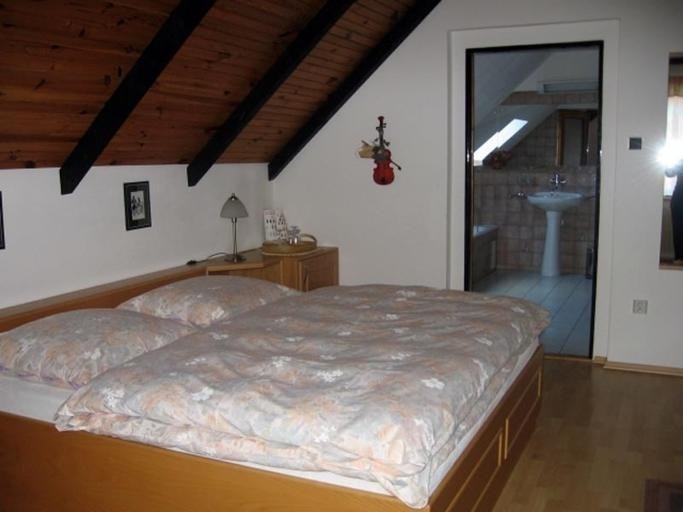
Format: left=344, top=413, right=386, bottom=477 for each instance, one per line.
left=527, top=190, right=583, bottom=212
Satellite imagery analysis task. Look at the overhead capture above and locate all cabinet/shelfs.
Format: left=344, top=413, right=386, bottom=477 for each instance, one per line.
left=206, top=244, right=339, bottom=292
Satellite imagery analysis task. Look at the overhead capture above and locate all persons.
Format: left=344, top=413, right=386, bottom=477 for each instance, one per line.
left=665, top=153, right=683, bottom=265
left=131, top=194, right=144, bottom=217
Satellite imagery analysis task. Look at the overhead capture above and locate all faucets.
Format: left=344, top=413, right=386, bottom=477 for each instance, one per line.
left=555, top=174, right=560, bottom=187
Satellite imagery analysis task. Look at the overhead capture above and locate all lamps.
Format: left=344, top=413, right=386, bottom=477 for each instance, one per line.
left=219, top=192, right=248, bottom=263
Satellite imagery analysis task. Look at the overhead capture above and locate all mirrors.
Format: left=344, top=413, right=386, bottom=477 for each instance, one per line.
left=657, top=51, right=683, bottom=270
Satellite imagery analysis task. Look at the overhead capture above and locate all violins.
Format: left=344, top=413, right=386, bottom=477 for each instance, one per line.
left=373, top=116, right=394, bottom=184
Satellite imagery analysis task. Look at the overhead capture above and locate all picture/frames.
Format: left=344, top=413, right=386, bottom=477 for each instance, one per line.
left=123, top=181, right=152, bottom=230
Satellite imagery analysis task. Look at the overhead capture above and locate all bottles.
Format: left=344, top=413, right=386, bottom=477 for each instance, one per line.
left=287, top=229, right=301, bottom=246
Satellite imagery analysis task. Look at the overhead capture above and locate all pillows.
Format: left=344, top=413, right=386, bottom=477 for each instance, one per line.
left=117, top=277, right=298, bottom=331
left=0, top=303, right=200, bottom=390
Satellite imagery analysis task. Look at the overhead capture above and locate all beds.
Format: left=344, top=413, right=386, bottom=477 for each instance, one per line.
left=0, top=274, right=553, bottom=512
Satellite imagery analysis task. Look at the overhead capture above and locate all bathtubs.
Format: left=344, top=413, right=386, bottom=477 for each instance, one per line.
left=472, top=225, right=498, bottom=239
